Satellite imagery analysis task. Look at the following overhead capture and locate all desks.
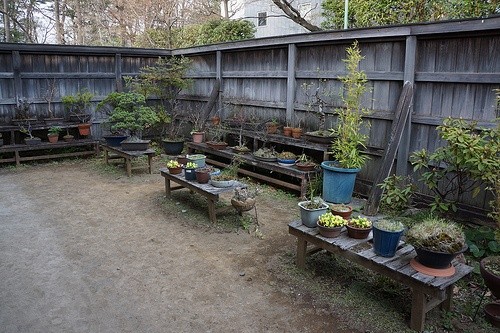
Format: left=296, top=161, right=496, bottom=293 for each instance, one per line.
left=287, top=219, right=475, bottom=333
left=161, top=168, right=247, bottom=220
left=102, top=143, right=156, bottom=176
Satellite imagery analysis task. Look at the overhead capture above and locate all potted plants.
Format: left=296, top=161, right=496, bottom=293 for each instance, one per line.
left=480, top=255, right=500, bottom=299
left=96, top=40, right=468, bottom=268
left=19, top=87, right=95, bottom=145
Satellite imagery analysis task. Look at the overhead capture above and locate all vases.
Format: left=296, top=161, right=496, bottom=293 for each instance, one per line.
left=44, top=118, right=64, bottom=125
left=70, top=114, right=92, bottom=122
left=12, top=119, right=37, bottom=126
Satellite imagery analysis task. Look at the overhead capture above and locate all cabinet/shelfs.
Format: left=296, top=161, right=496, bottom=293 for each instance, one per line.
left=187, top=126, right=336, bottom=198
left=0, top=122, right=99, bottom=165
